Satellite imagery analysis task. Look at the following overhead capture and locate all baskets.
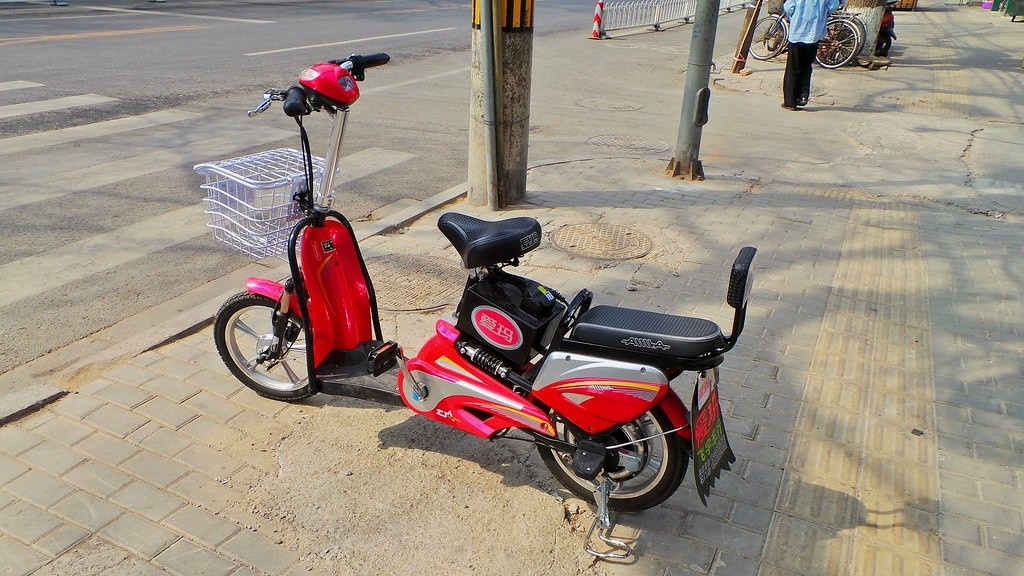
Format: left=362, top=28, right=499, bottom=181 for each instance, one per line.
left=194, top=148, right=341, bottom=261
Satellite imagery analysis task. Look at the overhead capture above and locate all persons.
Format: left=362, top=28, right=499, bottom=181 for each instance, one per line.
left=781, top=0, right=839, bottom=111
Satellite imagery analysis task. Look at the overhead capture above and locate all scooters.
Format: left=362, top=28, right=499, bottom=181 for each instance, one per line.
left=193, top=52, right=758, bottom=558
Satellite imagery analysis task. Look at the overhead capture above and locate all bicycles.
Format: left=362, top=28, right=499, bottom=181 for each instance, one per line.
left=749, top=3, right=868, bottom=70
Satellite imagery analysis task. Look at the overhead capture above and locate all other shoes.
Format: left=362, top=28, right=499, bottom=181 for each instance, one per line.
left=796, top=96, right=807, bottom=106
left=781, top=102, right=796, bottom=110
